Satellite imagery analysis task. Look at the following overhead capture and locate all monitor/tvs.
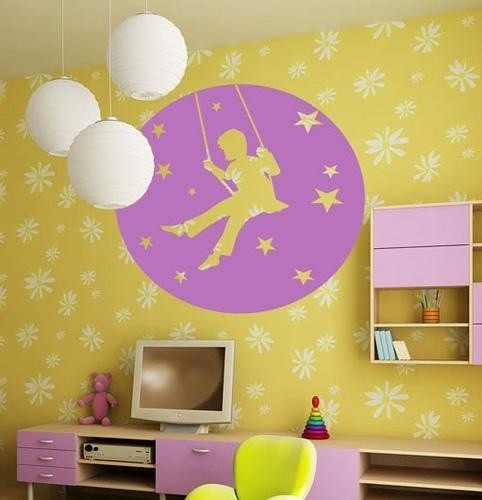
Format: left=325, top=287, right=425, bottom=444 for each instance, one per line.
left=130, top=339, right=235, bottom=435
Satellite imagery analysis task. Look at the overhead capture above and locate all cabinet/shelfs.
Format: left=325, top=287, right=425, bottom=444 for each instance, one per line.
left=369, top=201, right=481, bottom=366
left=16, top=422, right=481, bottom=499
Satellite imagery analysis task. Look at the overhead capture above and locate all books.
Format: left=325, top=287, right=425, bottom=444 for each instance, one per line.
left=374, top=329, right=412, bottom=360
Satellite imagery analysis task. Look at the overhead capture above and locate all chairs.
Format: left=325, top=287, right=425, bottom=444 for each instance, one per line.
left=184, top=435, right=317, bottom=500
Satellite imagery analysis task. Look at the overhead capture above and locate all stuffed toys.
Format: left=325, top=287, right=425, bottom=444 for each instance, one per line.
left=77, top=372, right=118, bottom=425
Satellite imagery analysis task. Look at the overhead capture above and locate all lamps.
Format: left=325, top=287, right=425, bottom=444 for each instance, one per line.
left=103, top=0, right=190, bottom=100
left=66, top=1, right=156, bottom=210
left=24, top=0, right=102, bottom=156
